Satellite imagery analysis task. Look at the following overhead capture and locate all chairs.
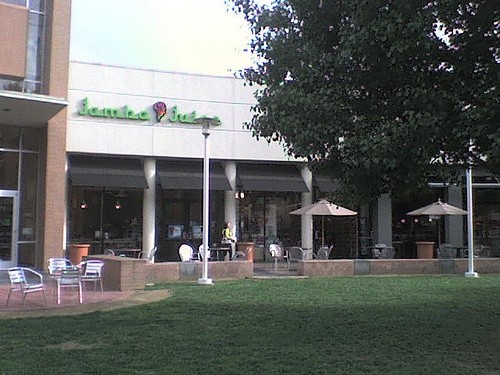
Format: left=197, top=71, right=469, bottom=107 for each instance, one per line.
left=46, top=257, right=105, bottom=305
left=288, top=247, right=308, bottom=271
left=104, top=246, right=158, bottom=264
left=313, top=245, right=334, bottom=259
left=179, top=244, right=200, bottom=262
left=436, top=244, right=455, bottom=258
left=268, top=244, right=289, bottom=270
left=5, top=267, right=47, bottom=309
left=198, top=245, right=214, bottom=261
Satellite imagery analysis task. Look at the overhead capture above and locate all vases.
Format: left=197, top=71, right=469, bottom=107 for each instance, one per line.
left=69, top=244, right=91, bottom=265
left=416, top=241, right=435, bottom=259
left=237, top=242, right=254, bottom=264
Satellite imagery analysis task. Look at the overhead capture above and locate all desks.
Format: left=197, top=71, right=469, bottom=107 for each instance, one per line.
left=444, top=247, right=467, bottom=258
left=285, top=247, right=311, bottom=259
left=209, top=248, right=230, bottom=261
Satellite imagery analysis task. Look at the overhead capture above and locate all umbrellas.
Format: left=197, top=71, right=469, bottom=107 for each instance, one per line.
left=406, top=200, right=469, bottom=248
left=288, top=198, right=358, bottom=246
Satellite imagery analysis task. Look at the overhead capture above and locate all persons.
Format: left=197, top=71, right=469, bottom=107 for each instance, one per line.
left=222, top=222, right=235, bottom=261
